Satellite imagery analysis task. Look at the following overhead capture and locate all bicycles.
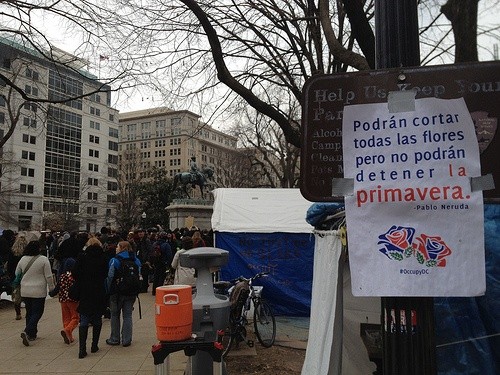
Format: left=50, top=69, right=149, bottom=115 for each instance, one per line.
left=222, top=271, right=277, bottom=361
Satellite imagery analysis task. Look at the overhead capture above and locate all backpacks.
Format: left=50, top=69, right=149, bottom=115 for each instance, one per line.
left=109, top=255, right=143, bottom=298
left=152, top=241, right=164, bottom=257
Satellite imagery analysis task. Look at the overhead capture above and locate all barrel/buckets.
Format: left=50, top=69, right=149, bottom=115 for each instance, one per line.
left=155, top=285, right=193, bottom=341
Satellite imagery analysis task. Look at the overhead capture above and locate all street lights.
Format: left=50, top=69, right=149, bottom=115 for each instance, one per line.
left=141, top=211, right=147, bottom=229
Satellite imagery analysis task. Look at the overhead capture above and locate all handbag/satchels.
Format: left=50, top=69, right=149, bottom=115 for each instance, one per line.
left=11, top=268, right=23, bottom=289
left=68, top=285, right=82, bottom=300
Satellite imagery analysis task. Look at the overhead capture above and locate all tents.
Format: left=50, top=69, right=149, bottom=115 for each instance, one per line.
left=209, top=188, right=315, bottom=317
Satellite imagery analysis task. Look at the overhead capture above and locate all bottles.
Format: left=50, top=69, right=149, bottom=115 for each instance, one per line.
left=199, top=305, right=214, bottom=338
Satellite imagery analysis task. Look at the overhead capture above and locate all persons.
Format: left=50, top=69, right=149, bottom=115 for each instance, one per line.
left=0, top=223, right=214, bottom=358
left=190, top=156, right=204, bottom=186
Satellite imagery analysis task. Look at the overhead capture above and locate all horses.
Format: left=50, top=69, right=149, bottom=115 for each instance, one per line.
left=171, top=167, right=214, bottom=199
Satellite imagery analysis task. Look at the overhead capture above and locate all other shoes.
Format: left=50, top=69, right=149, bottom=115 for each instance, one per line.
left=79, top=352, right=87, bottom=358
left=91, top=346, right=98, bottom=353
left=21, top=332, right=29, bottom=346
left=28, top=336, right=36, bottom=341
left=16, top=315, right=21, bottom=320
left=106, top=340, right=120, bottom=345
left=152, top=291, right=155, bottom=295
left=140, top=289, right=148, bottom=293
left=61, top=330, right=74, bottom=344
left=123, top=342, right=131, bottom=347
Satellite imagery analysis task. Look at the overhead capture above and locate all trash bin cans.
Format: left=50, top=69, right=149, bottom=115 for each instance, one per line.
left=178, top=245, right=232, bottom=356
